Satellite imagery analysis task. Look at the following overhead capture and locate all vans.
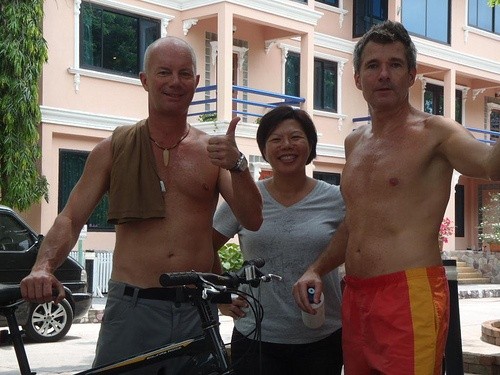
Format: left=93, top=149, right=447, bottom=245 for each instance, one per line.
left=0, top=205, right=94, bottom=343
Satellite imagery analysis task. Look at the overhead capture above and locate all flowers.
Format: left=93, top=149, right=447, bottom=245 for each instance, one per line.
left=438, top=218, right=455, bottom=243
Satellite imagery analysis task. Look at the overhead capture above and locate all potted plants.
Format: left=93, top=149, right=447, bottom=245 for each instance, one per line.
left=474, top=192, right=500, bottom=252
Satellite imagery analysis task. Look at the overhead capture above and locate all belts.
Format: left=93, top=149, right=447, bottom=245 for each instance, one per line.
left=123, top=286, right=203, bottom=303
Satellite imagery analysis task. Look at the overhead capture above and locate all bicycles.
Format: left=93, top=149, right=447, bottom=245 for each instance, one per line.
left=0, top=256, right=283, bottom=375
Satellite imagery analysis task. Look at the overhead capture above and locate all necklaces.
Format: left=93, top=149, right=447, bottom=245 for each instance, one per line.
left=148, top=123, right=192, bottom=166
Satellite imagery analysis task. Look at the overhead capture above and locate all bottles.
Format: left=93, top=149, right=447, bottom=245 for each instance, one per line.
left=301, top=288, right=325, bottom=329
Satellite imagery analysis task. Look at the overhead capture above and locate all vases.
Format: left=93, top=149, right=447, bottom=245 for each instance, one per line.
left=438, top=241, right=443, bottom=251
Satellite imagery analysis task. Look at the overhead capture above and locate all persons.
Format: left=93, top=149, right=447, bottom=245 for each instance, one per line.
left=213, top=106, right=346, bottom=375
left=292, top=20, right=500, bottom=375
left=20, top=35, right=263, bottom=375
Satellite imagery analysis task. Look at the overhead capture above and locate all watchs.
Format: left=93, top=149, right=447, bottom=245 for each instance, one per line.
left=226, top=151, right=249, bottom=174
